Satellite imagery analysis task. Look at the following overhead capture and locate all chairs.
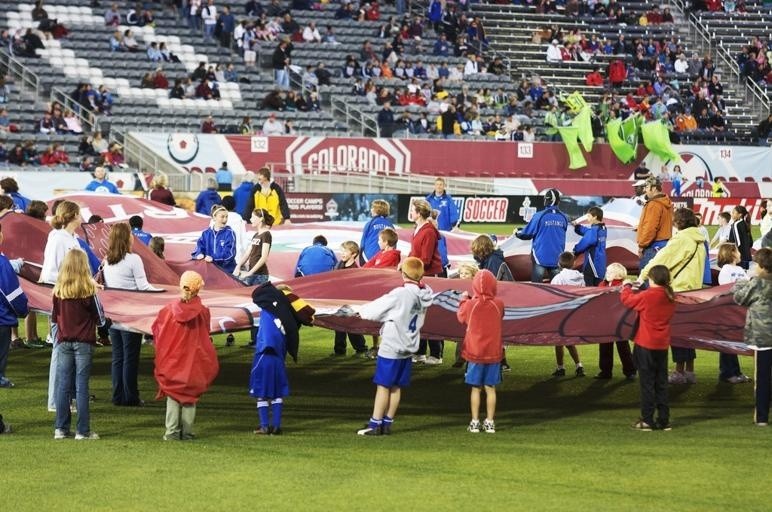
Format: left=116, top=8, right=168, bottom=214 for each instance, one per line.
left=2, top=1, right=772, bottom=146
left=1, top=71, right=143, bottom=176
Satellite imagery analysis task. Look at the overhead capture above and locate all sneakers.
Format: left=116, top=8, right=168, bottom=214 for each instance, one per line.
left=357, top=423, right=390, bottom=435
left=253, top=425, right=281, bottom=435
left=48, top=393, right=95, bottom=413
left=468, top=418, right=495, bottom=433
left=654, top=421, right=673, bottom=431
left=594, top=371, right=612, bottom=379
left=241, top=340, right=257, bottom=349
left=452, top=361, right=463, bottom=368
left=226, top=334, right=235, bottom=346
left=576, top=367, right=586, bottom=376
left=417, top=354, right=426, bottom=361
left=668, top=370, right=696, bottom=384
left=628, top=372, right=635, bottom=380
left=127, top=398, right=144, bottom=406
left=54, top=428, right=74, bottom=439
left=631, top=421, right=653, bottom=432
left=726, top=374, right=752, bottom=384
left=76, top=430, right=101, bottom=440
left=163, top=432, right=196, bottom=440
left=10, top=337, right=47, bottom=349
left=425, top=356, right=442, bottom=364
left=552, top=369, right=565, bottom=377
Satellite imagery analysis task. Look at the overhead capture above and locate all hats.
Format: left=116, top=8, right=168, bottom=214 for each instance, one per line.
left=544, top=189, right=558, bottom=200
left=180, top=271, right=202, bottom=289
left=632, top=177, right=660, bottom=187
